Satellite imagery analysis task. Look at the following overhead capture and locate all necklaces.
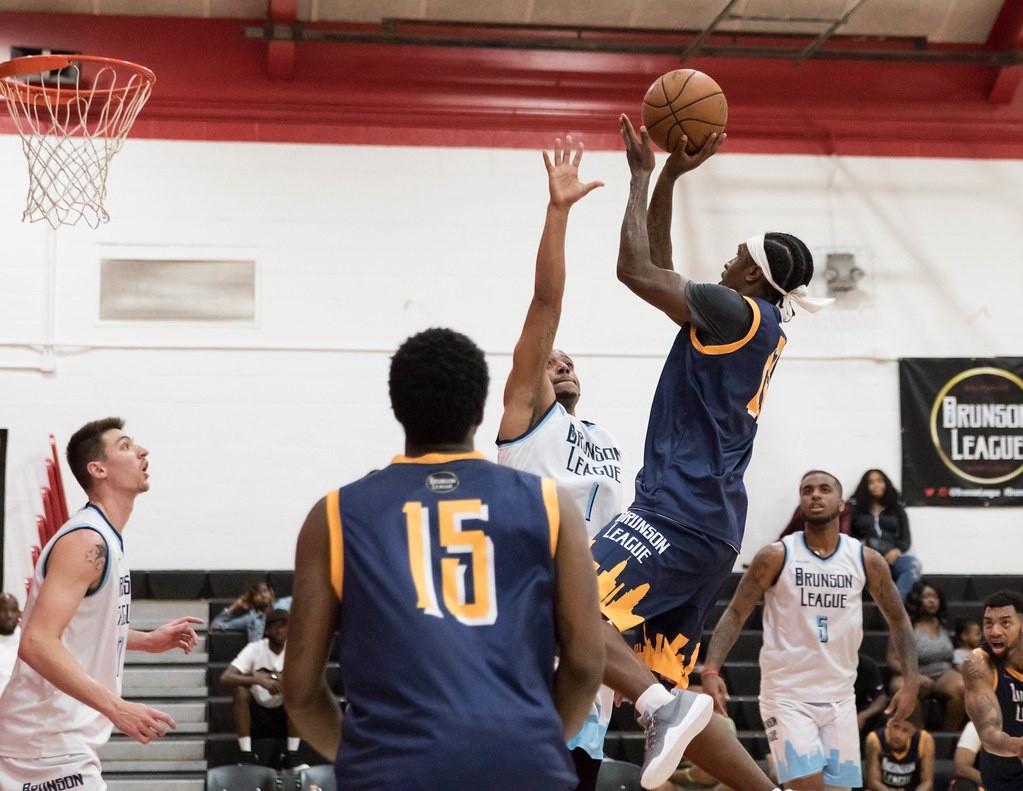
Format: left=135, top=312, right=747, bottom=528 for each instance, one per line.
left=814, top=550, right=820, bottom=555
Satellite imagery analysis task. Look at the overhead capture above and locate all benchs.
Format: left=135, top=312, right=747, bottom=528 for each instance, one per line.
left=95, top=568, right=1023, bottom=791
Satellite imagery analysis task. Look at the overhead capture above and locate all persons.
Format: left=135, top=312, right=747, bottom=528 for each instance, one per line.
left=589, top=114, right=814, bottom=791
left=219, top=606, right=312, bottom=779
left=647, top=673, right=738, bottom=791
left=961, top=591, right=1023, bottom=791
left=0, top=417, right=204, bottom=791
left=699, top=470, right=919, bottom=791
left=281, top=330, right=607, bottom=791
left=210, top=579, right=293, bottom=641
left=495, top=135, right=624, bottom=791
left=846, top=470, right=985, bottom=791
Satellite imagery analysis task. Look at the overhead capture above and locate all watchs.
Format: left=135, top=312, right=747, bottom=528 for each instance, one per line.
left=271, top=673, right=278, bottom=679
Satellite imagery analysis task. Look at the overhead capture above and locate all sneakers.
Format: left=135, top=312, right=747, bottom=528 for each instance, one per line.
left=638, top=687, right=714, bottom=789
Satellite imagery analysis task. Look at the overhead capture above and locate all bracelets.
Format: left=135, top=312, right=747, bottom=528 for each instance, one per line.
left=700, top=671, right=718, bottom=678
left=684, top=768, right=695, bottom=783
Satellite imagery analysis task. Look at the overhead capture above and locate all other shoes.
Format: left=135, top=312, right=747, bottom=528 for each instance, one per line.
left=278, top=751, right=310, bottom=775
left=237, top=752, right=261, bottom=766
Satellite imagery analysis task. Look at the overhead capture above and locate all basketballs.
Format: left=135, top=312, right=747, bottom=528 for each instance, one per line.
left=641, top=68, right=728, bottom=154
